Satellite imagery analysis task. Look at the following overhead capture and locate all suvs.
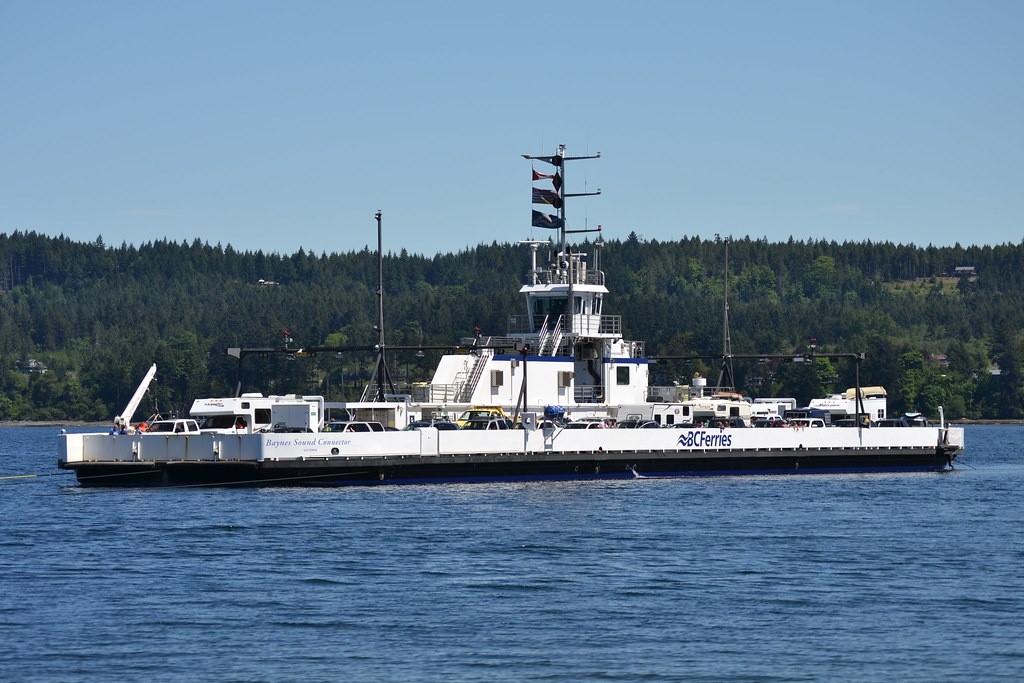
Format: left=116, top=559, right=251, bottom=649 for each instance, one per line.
left=708, top=416, right=746, bottom=429
left=321, top=420, right=386, bottom=434
left=138, top=418, right=202, bottom=435
left=457, top=415, right=514, bottom=429
left=399, top=416, right=460, bottom=431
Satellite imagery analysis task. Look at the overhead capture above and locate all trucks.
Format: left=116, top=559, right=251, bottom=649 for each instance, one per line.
left=271, top=401, right=319, bottom=434
left=189, top=393, right=325, bottom=435
left=456, top=405, right=522, bottom=429
left=614, top=402, right=694, bottom=428
left=807, top=396, right=887, bottom=427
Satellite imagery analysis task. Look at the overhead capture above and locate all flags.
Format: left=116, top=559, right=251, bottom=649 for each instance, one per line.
left=532, top=187, right=556, bottom=204
left=533, top=170, right=555, bottom=181
left=532, top=209, right=562, bottom=229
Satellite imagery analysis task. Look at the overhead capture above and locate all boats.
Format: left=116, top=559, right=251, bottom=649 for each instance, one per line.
left=55, top=145, right=966, bottom=491
left=846, top=386, right=887, bottom=400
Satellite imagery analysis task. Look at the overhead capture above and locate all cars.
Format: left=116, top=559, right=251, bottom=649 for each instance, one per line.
left=755, top=419, right=785, bottom=428
left=876, top=418, right=910, bottom=428
left=788, top=418, right=826, bottom=428
left=514, top=420, right=558, bottom=430
left=750, top=414, right=782, bottom=427
left=565, top=421, right=605, bottom=430
left=833, top=418, right=867, bottom=428
left=273, top=425, right=311, bottom=434
left=614, top=420, right=660, bottom=428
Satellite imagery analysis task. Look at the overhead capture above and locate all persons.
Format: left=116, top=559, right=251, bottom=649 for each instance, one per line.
left=325, top=418, right=355, bottom=432
left=693, top=419, right=807, bottom=432
left=598, top=419, right=617, bottom=429
left=110, top=422, right=152, bottom=436
left=237, top=420, right=244, bottom=429
